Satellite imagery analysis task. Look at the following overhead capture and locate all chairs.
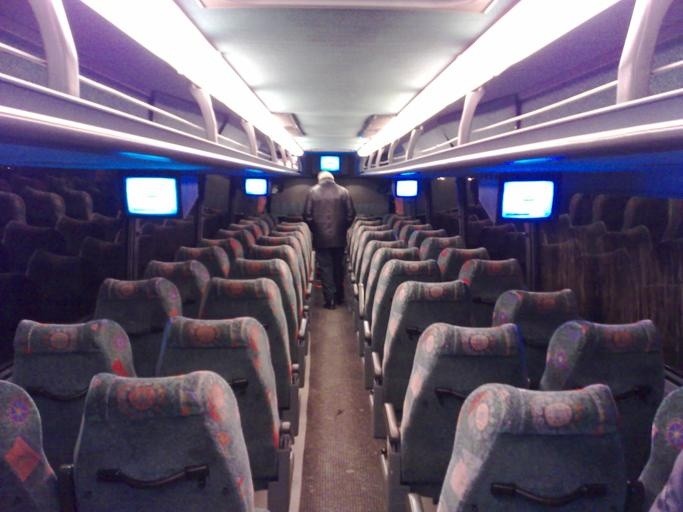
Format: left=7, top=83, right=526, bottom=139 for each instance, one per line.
left=1, top=172, right=207, bottom=344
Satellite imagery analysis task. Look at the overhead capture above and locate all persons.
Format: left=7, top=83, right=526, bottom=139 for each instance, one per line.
left=302, top=170, right=356, bottom=309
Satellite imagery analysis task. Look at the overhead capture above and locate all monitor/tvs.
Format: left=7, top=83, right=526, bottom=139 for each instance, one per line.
left=498, top=172, right=561, bottom=222
left=121, top=172, right=198, bottom=220
left=241, top=177, right=272, bottom=196
left=320, top=156, right=341, bottom=171
left=395, top=179, right=418, bottom=198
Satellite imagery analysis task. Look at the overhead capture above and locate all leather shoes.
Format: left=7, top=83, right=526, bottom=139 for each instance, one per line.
left=324, top=299, right=335, bottom=309
